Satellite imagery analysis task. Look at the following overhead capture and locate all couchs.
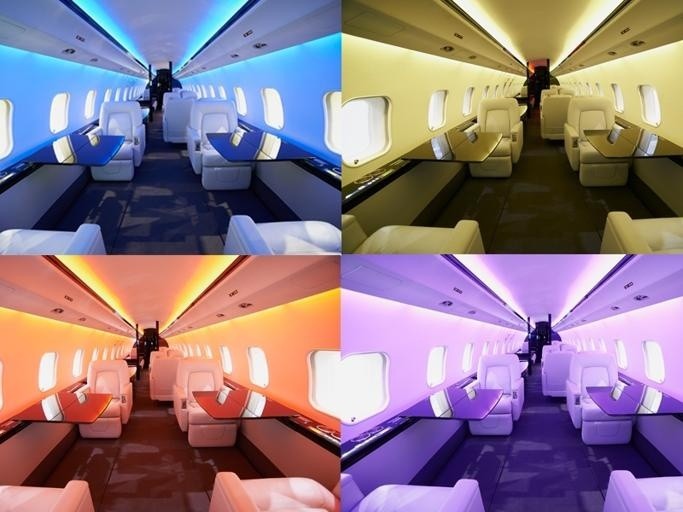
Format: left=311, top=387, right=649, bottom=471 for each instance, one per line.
left=0, top=480, right=95, bottom=512
left=0, top=224, right=107, bottom=255
left=541, top=344, right=560, bottom=364
left=563, top=97, right=633, bottom=187
left=149, top=357, right=180, bottom=401
left=542, top=352, right=573, bottom=398
left=467, top=353, right=524, bottom=436
left=185, top=101, right=255, bottom=191
left=468, top=98, right=524, bottom=178
left=75, top=360, right=133, bottom=438
left=341, top=215, right=486, bottom=255
left=167, top=350, right=183, bottom=358
left=602, top=211, right=683, bottom=252
left=149, top=351, right=167, bottom=369
left=340, top=472, right=486, bottom=512
left=162, top=91, right=181, bottom=110
left=162, top=98, right=195, bottom=144
left=560, top=344, right=577, bottom=352
left=209, top=471, right=340, bottom=512
left=559, top=88, right=576, bottom=96
left=540, top=89, right=559, bottom=105
left=539, top=95, right=572, bottom=141
left=566, top=352, right=637, bottom=445
left=604, top=470, right=682, bottom=512
left=223, top=214, right=341, bottom=255
left=181, top=91, right=197, bottom=99
left=86, top=100, right=146, bottom=182
left=172, top=359, right=240, bottom=447
